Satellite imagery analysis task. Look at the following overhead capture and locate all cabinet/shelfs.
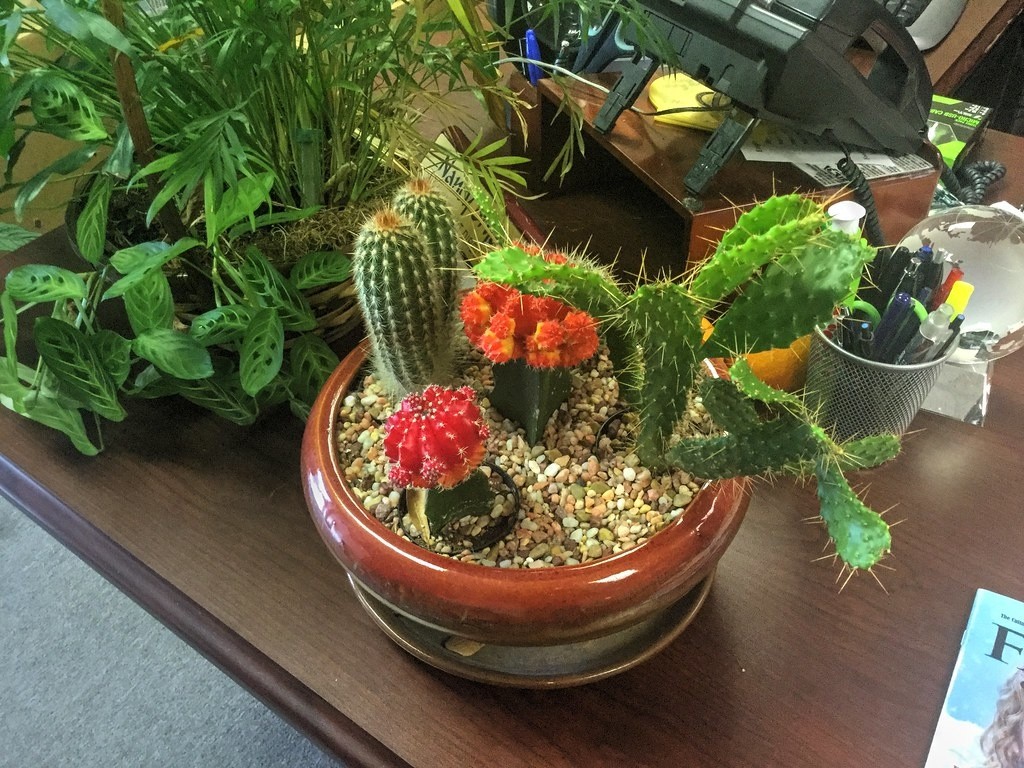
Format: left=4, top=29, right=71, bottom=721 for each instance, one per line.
left=539, top=68, right=937, bottom=282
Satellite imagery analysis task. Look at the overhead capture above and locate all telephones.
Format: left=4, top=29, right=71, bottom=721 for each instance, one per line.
left=615, top=0, right=934, bottom=158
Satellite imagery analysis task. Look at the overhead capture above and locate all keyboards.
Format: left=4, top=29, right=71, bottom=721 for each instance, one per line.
left=873, top=0, right=967, bottom=51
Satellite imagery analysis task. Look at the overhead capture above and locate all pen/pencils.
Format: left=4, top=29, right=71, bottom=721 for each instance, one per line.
left=507, top=25, right=571, bottom=88
left=834, top=243, right=974, bottom=366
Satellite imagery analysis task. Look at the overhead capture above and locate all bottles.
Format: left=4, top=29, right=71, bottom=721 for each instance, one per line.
left=825, top=198, right=868, bottom=317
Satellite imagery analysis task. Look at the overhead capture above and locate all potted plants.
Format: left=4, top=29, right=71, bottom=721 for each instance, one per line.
left=308, top=169, right=931, bottom=647
left=1, top=0, right=622, bottom=459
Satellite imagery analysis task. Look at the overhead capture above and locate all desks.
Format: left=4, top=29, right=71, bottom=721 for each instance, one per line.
left=0, top=0, right=1024, bottom=768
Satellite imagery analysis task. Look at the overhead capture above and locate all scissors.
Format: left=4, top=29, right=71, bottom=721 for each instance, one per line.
left=839, top=294, right=929, bottom=334
